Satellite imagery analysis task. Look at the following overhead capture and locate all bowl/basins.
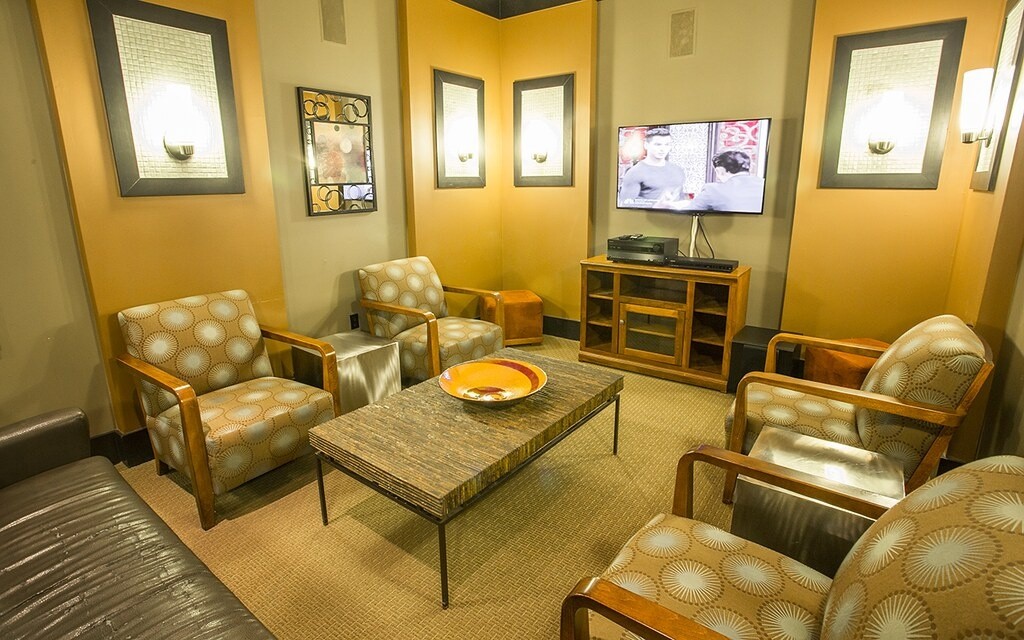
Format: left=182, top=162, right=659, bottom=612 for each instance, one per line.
left=438, top=358, right=548, bottom=407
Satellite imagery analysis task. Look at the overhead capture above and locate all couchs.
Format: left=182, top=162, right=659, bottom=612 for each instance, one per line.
left=0, top=411, right=305, bottom=640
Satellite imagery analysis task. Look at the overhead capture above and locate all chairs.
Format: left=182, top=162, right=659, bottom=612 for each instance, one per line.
left=119, top=290, right=339, bottom=529
left=721, top=314, right=992, bottom=521
left=356, top=255, right=502, bottom=379
left=561, top=445, right=1024, bottom=640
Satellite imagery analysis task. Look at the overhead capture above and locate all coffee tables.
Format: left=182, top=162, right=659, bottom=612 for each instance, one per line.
left=309, top=348, right=622, bottom=606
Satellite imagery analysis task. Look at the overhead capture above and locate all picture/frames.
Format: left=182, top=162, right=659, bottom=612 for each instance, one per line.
left=512, top=73, right=575, bottom=186
left=298, top=87, right=382, bottom=217
left=974, top=0, right=1024, bottom=190
left=86, top=1, right=246, bottom=197
left=816, top=14, right=969, bottom=190
left=432, top=69, right=487, bottom=189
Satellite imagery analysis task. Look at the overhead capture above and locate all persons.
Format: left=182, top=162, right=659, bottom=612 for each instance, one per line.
left=619, top=128, right=762, bottom=213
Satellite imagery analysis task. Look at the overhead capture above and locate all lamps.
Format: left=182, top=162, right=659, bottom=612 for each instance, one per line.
left=161, top=128, right=195, bottom=160
left=868, top=133, right=896, bottom=155
left=961, top=68, right=995, bottom=146
left=458, top=147, right=473, bottom=163
left=532, top=151, right=548, bottom=163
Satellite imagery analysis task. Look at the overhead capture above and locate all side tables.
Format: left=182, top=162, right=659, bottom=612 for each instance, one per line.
left=292, top=331, right=403, bottom=416
left=733, top=425, right=906, bottom=580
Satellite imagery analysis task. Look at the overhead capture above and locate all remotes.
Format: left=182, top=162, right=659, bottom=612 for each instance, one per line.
left=619, top=232, right=643, bottom=239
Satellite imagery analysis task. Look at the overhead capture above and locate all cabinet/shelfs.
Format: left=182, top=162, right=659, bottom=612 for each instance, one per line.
left=579, top=254, right=749, bottom=391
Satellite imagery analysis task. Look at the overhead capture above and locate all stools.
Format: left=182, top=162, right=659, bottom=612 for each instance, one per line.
left=804, top=338, right=891, bottom=386
left=481, top=291, right=544, bottom=340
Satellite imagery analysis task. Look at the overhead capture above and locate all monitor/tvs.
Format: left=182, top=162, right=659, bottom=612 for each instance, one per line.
left=615, top=118, right=772, bottom=216
